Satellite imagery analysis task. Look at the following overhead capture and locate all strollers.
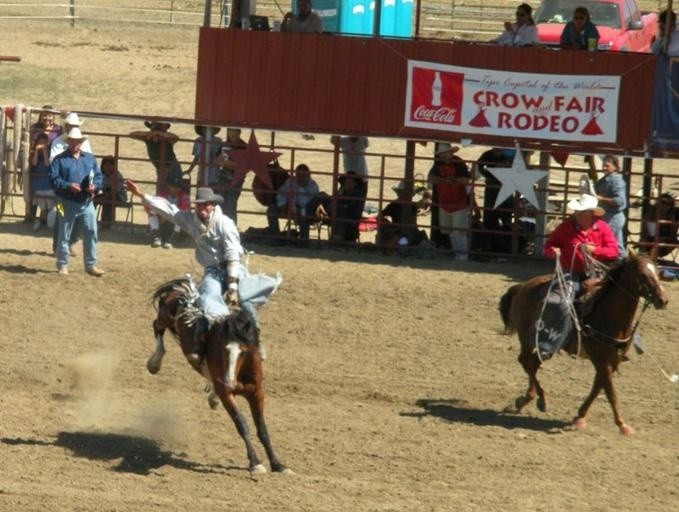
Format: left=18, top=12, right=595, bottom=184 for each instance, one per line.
left=516, top=187, right=564, bottom=256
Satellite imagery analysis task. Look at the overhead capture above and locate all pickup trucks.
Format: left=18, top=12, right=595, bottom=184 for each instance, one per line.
left=532, top=1, right=659, bottom=54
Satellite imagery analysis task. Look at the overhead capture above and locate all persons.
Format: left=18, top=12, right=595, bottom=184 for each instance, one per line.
left=648, top=9, right=678, bottom=57
left=558, top=7, right=600, bottom=52
left=278, top=0, right=325, bottom=36
left=428, top=143, right=471, bottom=262
left=326, top=135, right=368, bottom=244
left=477, top=148, right=516, bottom=231
left=649, top=192, right=678, bottom=259
left=490, top=4, right=540, bottom=47
left=129, top=120, right=191, bottom=247
left=182, top=124, right=249, bottom=225
left=120, top=173, right=268, bottom=367
left=536, top=193, right=647, bottom=361
left=377, top=181, right=418, bottom=247
left=101, top=155, right=128, bottom=227
left=596, top=156, right=627, bottom=257
left=267, top=163, right=321, bottom=240
left=26, top=105, right=106, bottom=277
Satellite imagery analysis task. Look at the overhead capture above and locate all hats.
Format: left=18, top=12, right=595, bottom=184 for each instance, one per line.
left=391, top=180, right=415, bottom=196
left=191, top=186, right=224, bottom=206
left=62, top=127, right=87, bottom=143
left=62, top=113, right=85, bottom=125
left=339, top=170, right=363, bottom=185
left=567, top=193, right=606, bottom=217
left=194, top=124, right=221, bottom=136
left=433, top=141, right=458, bottom=154
left=144, top=120, right=171, bottom=130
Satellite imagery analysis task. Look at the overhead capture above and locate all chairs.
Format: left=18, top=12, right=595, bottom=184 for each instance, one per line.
left=97, top=176, right=136, bottom=236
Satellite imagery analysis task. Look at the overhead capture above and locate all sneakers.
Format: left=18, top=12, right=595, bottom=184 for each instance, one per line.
left=151, top=229, right=164, bottom=247
left=163, top=231, right=178, bottom=249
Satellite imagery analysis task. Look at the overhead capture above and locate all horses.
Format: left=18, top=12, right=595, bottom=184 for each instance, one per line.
left=147, top=279, right=295, bottom=475
left=499, top=245, right=670, bottom=435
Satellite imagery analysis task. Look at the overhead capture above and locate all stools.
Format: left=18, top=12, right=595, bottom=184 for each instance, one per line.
left=318, top=223, right=361, bottom=244
left=279, top=216, right=321, bottom=238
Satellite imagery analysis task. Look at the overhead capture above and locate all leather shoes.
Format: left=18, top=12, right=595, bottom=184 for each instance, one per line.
left=88, top=266, right=105, bottom=276
left=58, top=267, right=69, bottom=275
